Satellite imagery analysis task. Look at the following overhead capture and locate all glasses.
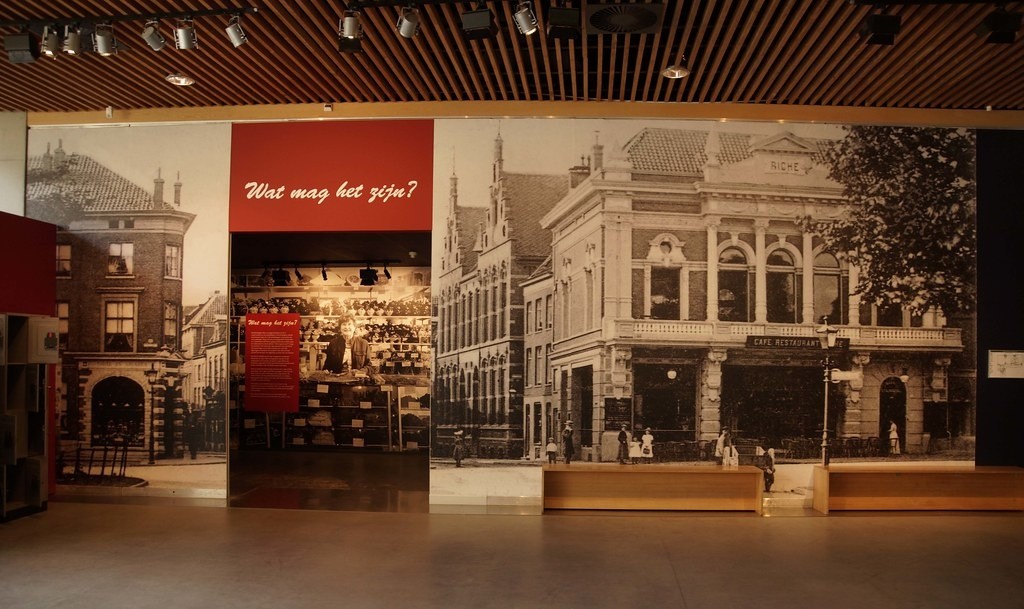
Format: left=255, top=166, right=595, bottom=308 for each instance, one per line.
left=341, top=323, right=355, bottom=327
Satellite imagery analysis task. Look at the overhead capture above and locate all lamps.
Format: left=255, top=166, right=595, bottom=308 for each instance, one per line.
left=38, top=24, right=60, bottom=56
left=394, top=6, right=420, bottom=40
left=61, top=22, right=83, bottom=56
left=461, top=7, right=501, bottom=39
left=337, top=7, right=363, bottom=40
left=857, top=13, right=904, bottom=47
left=166, top=71, right=197, bottom=87
left=662, top=65, right=691, bottom=79
left=977, top=8, right=1023, bottom=47
left=667, top=363, right=677, bottom=380
left=901, top=366, right=909, bottom=383
left=140, top=19, right=167, bottom=52
left=3, top=29, right=42, bottom=64
left=171, top=19, right=199, bottom=50
left=511, top=1, right=540, bottom=36
left=224, top=15, right=249, bottom=49
left=89, top=22, right=118, bottom=58
left=545, top=6, right=582, bottom=39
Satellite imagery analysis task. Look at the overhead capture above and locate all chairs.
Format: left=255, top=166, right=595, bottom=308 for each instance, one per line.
left=652, top=435, right=895, bottom=463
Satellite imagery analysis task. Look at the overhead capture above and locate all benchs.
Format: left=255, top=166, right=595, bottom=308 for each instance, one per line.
left=812, top=464, right=1024, bottom=515
left=540, top=463, right=764, bottom=517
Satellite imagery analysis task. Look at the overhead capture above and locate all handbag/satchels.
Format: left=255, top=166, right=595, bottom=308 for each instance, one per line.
left=643, top=446, right=651, bottom=454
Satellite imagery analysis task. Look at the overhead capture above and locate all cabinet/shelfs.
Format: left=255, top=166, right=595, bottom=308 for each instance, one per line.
left=230, top=270, right=430, bottom=454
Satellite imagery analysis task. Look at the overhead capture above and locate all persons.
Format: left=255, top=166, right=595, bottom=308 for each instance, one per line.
left=453, top=438, right=465, bottom=467
left=629, top=436, right=642, bottom=464
left=640, top=428, right=654, bottom=464
left=561, top=420, right=575, bottom=465
left=186, top=424, right=200, bottom=459
left=758, top=447, right=776, bottom=493
left=715, top=428, right=726, bottom=465
left=546, top=437, right=559, bottom=464
left=616, top=424, right=629, bottom=465
left=320, top=313, right=373, bottom=402
left=889, top=419, right=902, bottom=456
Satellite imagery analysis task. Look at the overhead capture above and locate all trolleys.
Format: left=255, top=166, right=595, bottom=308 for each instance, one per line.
left=728, top=445, right=775, bottom=492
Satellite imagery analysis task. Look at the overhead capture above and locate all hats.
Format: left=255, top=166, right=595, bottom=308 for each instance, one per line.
left=453, top=430, right=464, bottom=436
left=548, top=437, right=555, bottom=440
left=644, top=427, right=652, bottom=432
left=563, top=420, right=577, bottom=426
left=621, top=423, right=628, bottom=427
left=721, top=426, right=733, bottom=431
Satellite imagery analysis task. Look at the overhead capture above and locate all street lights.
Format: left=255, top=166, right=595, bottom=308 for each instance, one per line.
left=811, top=313, right=839, bottom=467
